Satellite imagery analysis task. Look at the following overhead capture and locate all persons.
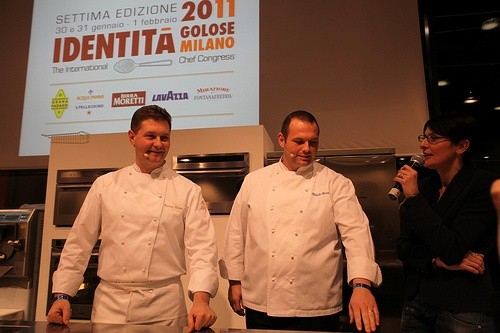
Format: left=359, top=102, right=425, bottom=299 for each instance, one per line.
left=45, top=105, right=219, bottom=332
left=218, top=110, right=382, bottom=333
left=394, top=114, right=500, bottom=333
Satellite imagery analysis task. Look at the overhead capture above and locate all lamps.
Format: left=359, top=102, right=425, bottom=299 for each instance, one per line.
left=481, top=17, right=500, bottom=30
left=463, top=90, right=479, bottom=106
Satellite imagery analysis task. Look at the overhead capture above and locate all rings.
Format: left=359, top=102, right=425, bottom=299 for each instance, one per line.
left=474, top=254, right=480, bottom=259
left=204, top=325, right=208, bottom=327
left=401, top=174, right=404, bottom=179
left=368, top=310, right=374, bottom=313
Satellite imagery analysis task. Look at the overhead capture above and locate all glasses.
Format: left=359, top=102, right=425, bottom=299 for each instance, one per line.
left=417, top=134, right=447, bottom=145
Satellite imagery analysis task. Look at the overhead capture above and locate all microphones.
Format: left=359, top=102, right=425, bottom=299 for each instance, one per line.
left=387, top=153, right=425, bottom=201
left=134, top=137, right=148, bottom=159
left=283, top=148, right=294, bottom=157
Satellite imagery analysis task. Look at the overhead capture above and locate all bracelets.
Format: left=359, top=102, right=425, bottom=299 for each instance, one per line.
left=431, top=255, right=441, bottom=268
left=352, top=283, right=373, bottom=292
left=51, top=295, right=72, bottom=303
left=398, top=190, right=419, bottom=209
